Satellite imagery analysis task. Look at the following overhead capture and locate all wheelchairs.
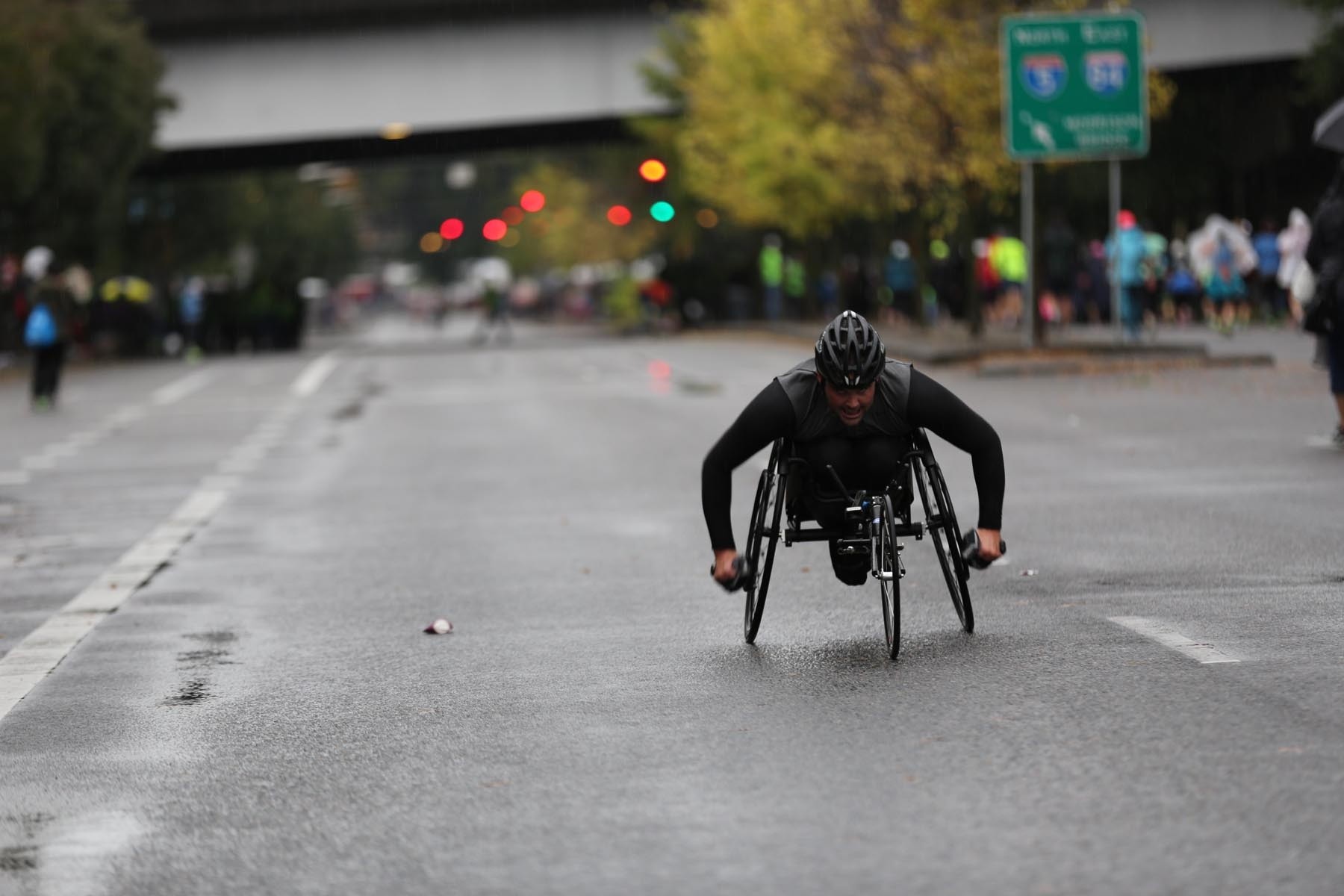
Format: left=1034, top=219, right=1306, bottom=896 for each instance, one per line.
left=721, top=427, right=1006, bottom=660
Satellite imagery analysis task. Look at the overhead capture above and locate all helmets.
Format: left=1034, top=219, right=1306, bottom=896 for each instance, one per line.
left=814, top=311, right=885, bottom=392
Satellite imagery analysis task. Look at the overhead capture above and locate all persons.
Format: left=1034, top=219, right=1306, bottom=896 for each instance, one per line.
left=701, top=310, right=1005, bottom=585
left=0, top=209, right=1316, bottom=415
left=1300, top=162, right=1344, bottom=441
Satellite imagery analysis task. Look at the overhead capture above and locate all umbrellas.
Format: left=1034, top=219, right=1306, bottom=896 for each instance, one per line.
left=1312, top=97, right=1344, bottom=151
